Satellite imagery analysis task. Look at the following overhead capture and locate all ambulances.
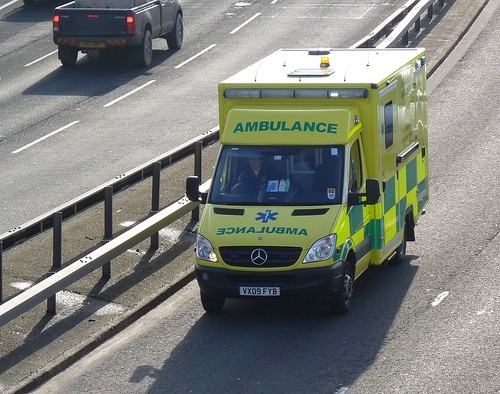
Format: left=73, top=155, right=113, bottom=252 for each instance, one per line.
left=184, top=48, right=431, bottom=315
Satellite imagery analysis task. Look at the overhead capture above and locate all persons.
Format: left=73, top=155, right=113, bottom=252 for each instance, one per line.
left=238, top=158, right=282, bottom=194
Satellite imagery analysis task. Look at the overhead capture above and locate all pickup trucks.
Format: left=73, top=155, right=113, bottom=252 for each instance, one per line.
left=52, top=0, right=184, bottom=67
